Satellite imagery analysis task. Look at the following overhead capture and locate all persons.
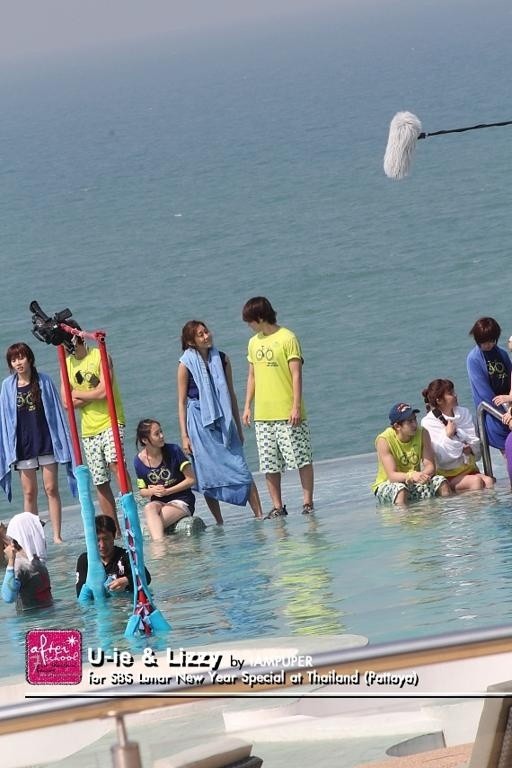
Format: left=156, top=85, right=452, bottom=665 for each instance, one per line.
left=241, top=296, right=314, bottom=519
left=420, top=379, right=493, bottom=491
left=133, top=418, right=196, bottom=539
left=76, top=515, right=152, bottom=598
left=493, top=336, right=512, bottom=489
left=371, top=404, right=455, bottom=504
left=1, top=342, right=77, bottom=544
left=0, top=512, right=57, bottom=614
left=177, top=321, right=262, bottom=525
left=60, top=319, right=133, bottom=540
left=466, top=318, right=512, bottom=450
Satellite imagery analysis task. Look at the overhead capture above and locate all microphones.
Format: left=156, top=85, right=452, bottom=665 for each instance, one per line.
left=432, top=407, right=459, bottom=437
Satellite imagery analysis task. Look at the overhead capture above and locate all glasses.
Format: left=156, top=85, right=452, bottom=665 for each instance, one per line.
left=480, top=337, right=496, bottom=342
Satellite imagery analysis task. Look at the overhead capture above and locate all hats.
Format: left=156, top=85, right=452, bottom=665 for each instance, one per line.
left=389, top=402, right=420, bottom=425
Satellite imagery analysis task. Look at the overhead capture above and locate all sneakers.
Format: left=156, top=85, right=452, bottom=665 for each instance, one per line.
left=263, top=504, right=288, bottom=520
left=302, top=502, right=314, bottom=513
